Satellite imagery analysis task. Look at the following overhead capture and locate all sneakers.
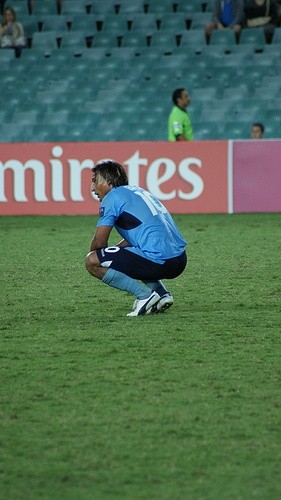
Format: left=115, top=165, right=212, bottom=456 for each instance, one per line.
left=145, top=292, right=175, bottom=315
left=126, top=290, right=161, bottom=316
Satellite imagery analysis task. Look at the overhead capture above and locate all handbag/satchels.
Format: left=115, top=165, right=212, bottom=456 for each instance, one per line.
left=0, top=24, right=25, bottom=47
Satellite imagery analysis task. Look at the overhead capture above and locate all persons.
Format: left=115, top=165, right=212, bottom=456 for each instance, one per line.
left=85, top=161, right=188, bottom=317
left=168, top=87, right=194, bottom=143
left=0, top=6, right=25, bottom=48
left=251, top=123, right=264, bottom=139
left=205, top=0, right=243, bottom=35
left=239, top=0, right=278, bottom=45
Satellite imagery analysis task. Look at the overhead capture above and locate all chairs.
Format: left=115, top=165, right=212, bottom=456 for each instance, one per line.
left=0, top=0, right=281, bottom=142
